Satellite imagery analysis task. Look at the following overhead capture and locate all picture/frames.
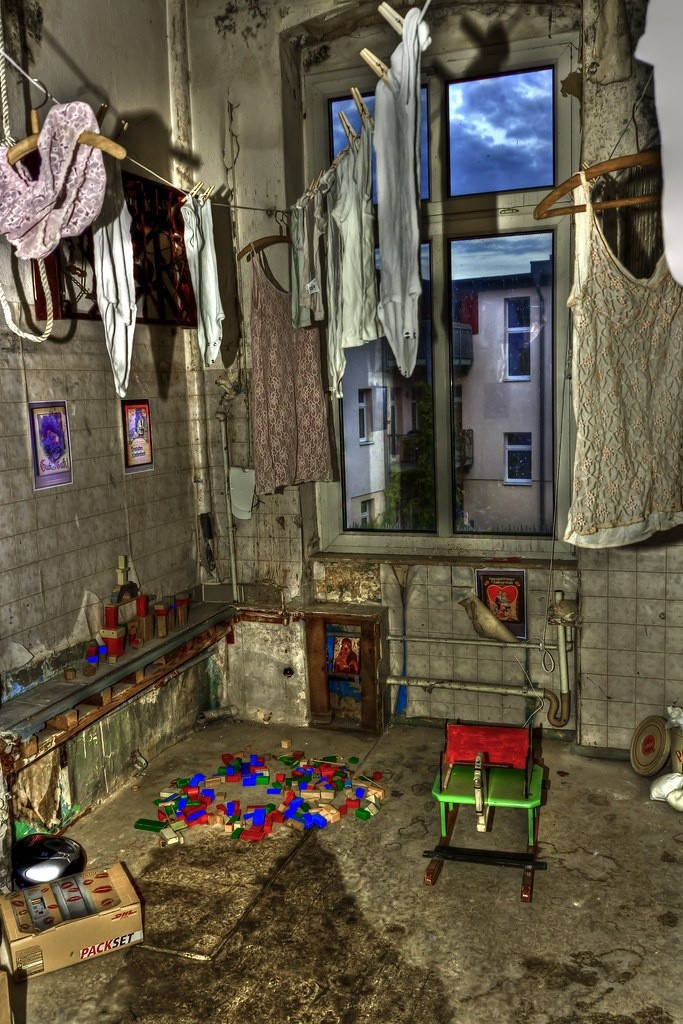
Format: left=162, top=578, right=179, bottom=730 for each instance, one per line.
left=121, top=398, right=154, bottom=475
left=28, top=400, right=73, bottom=491
left=476, top=568, right=526, bottom=640
